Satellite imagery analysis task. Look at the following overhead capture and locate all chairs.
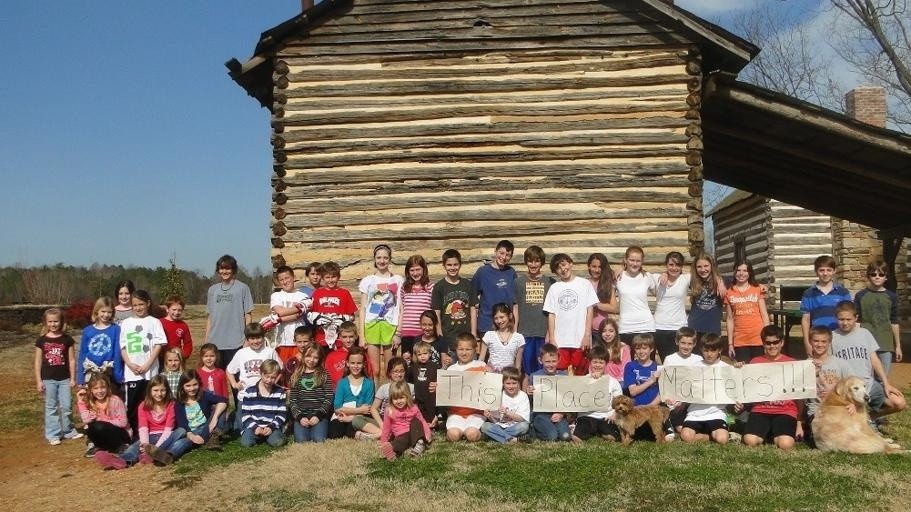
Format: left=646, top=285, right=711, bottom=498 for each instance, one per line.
left=772, top=284, right=811, bottom=353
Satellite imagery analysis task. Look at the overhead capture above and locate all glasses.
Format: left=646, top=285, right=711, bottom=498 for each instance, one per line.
left=869, top=273, right=886, bottom=277
left=762, top=339, right=781, bottom=345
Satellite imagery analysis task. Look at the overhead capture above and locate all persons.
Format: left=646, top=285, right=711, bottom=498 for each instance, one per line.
left=352, top=239, right=907, bottom=471
left=34, top=254, right=375, bottom=471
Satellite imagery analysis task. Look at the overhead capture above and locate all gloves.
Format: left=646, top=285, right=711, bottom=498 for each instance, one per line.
left=295, top=297, right=314, bottom=314
left=258, top=313, right=281, bottom=332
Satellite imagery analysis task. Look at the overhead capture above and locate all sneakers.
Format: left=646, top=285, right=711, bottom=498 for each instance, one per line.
left=381, top=442, right=396, bottom=462
left=140, top=443, right=174, bottom=466
left=85, top=447, right=128, bottom=470
left=71, top=433, right=84, bottom=441
left=354, top=430, right=376, bottom=441
left=867, top=420, right=880, bottom=434
left=50, top=440, right=62, bottom=447
left=206, top=434, right=223, bottom=451
left=408, top=437, right=426, bottom=460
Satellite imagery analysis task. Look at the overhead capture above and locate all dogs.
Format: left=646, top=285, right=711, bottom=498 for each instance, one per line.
left=810, top=375, right=911, bottom=456
left=610, top=393, right=671, bottom=446
